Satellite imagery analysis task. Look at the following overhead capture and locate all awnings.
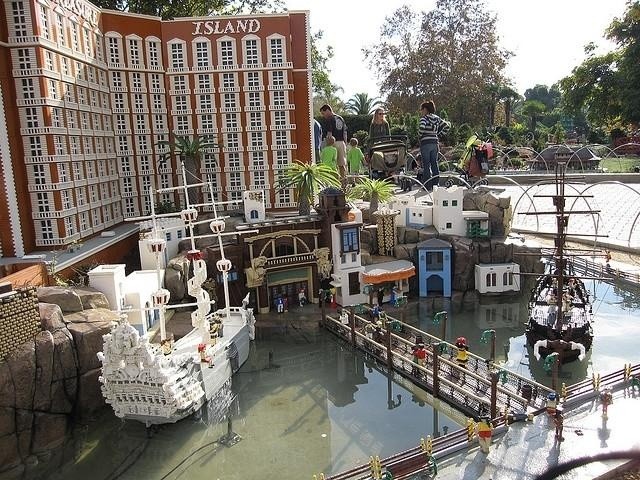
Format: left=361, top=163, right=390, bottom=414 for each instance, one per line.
left=361, top=260, right=415, bottom=284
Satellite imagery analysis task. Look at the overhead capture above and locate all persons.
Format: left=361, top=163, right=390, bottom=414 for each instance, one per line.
left=299, top=289, right=306, bottom=308
left=209, top=318, right=224, bottom=346
left=339, top=304, right=468, bottom=375
left=391, top=285, right=398, bottom=305
left=319, top=104, right=348, bottom=179
left=318, top=135, right=338, bottom=175
left=416, top=100, right=451, bottom=191
left=313, top=117, right=323, bottom=167
left=546, top=393, right=565, bottom=440
left=276, top=294, right=283, bottom=313
left=378, top=287, right=384, bottom=311
left=368, top=106, right=391, bottom=161
left=318, top=285, right=336, bottom=308
left=346, top=137, right=368, bottom=176
left=599, top=387, right=612, bottom=414
left=477, top=415, right=492, bottom=453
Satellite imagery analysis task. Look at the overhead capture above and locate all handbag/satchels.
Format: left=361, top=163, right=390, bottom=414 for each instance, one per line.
left=469, top=143, right=493, bottom=177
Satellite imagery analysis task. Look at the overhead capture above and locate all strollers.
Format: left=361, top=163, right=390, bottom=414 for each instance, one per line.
left=445, top=132, right=492, bottom=188
left=368, top=135, right=412, bottom=192
